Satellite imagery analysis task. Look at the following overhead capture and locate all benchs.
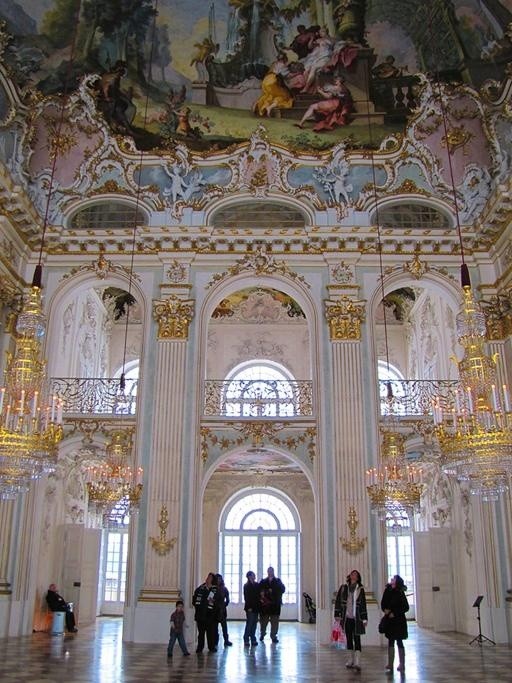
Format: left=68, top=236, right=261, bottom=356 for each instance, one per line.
left=31, top=609, right=66, bottom=635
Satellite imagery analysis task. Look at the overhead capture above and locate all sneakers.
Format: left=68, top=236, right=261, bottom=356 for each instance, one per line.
left=167, top=633, right=279, bottom=658
left=68, top=628, right=78, bottom=633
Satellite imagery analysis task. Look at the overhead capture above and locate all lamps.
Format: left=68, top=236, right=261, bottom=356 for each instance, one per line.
left=0, top=0, right=80, bottom=502
left=355, top=2, right=424, bottom=523
left=421, top=3, right=512, bottom=503
left=145, top=504, right=178, bottom=558
left=340, top=505, right=370, bottom=557
left=80, top=3, right=157, bottom=532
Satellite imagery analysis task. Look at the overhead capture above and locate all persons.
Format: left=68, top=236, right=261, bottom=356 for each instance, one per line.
left=251, top=22, right=399, bottom=133
left=214, top=573, right=232, bottom=646
left=191, top=572, right=221, bottom=654
left=47, top=584, right=78, bottom=632
left=259, top=566, right=285, bottom=642
left=103, top=58, right=140, bottom=137
left=167, top=601, right=191, bottom=657
left=380, top=574, right=409, bottom=670
left=243, top=570, right=259, bottom=645
left=173, top=105, right=198, bottom=137
left=333, top=569, right=368, bottom=671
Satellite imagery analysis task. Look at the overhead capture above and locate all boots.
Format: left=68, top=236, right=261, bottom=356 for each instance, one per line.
left=345, top=650, right=361, bottom=670
left=385, top=646, right=395, bottom=670
left=397, top=648, right=405, bottom=670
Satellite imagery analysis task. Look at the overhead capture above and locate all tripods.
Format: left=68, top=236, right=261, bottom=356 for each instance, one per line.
left=469, top=604, right=496, bottom=645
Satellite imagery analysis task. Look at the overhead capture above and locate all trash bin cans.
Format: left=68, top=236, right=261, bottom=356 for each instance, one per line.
left=49, top=611, right=65, bottom=635
